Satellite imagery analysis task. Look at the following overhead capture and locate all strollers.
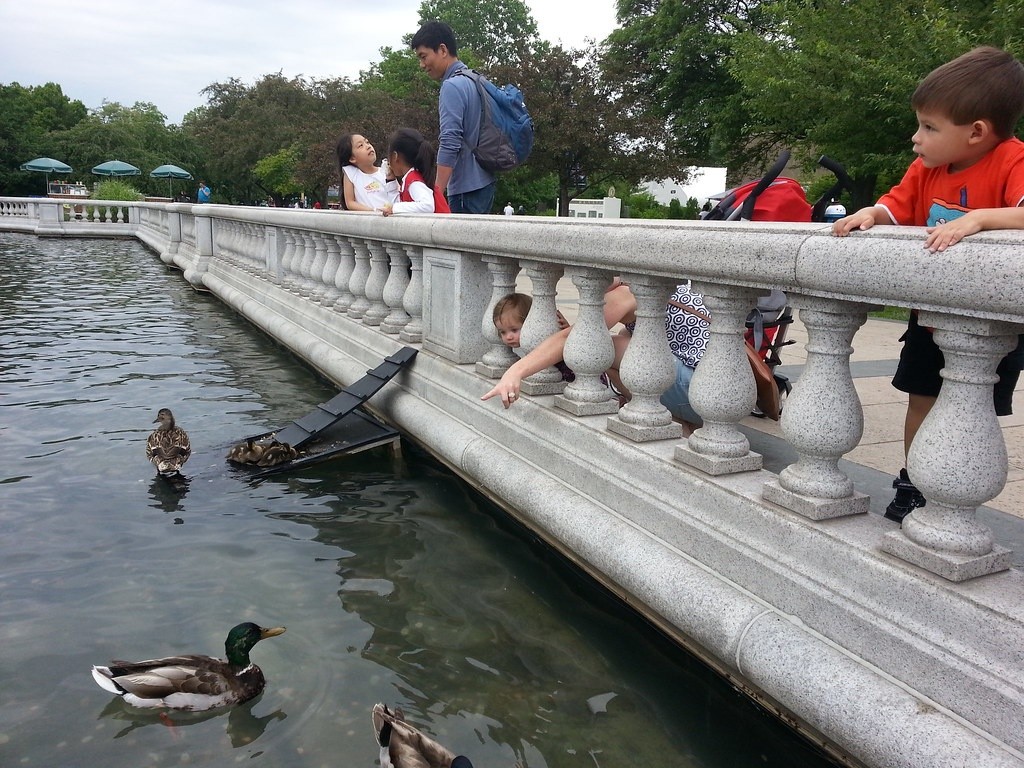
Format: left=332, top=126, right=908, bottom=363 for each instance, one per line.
left=691, top=148, right=858, bottom=418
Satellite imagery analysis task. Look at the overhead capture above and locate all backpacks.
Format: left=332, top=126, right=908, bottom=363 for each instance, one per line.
left=451, top=69, right=534, bottom=173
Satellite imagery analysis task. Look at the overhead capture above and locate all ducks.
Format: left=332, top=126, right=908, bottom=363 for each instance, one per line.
left=223, top=437, right=298, bottom=466
left=145, top=408, right=191, bottom=474
left=90, top=621, right=287, bottom=712
left=371, top=701, right=472, bottom=768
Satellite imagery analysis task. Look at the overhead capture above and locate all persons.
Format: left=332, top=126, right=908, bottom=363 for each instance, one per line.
left=294, top=200, right=305, bottom=209
left=699, top=198, right=713, bottom=220
left=504, top=202, right=514, bottom=215
left=830, top=43, right=1024, bottom=525
left=197, top=181, right=211, bottom=204
left=337, top=128, right=452, bottom=213
left=260, top=200, right=269, bottom=207
left=410, top=19, right=536, bottom=214
left=479, top=274, right=781, bottom=439
left=313, top=201, right=322, bottom=209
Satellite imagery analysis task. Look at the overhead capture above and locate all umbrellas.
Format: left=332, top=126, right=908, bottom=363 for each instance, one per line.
left=19, top=155, right=73, bottom=193
left=92, top=157, right=142, bottom=182
left=150, top=162, right=195, bottom=198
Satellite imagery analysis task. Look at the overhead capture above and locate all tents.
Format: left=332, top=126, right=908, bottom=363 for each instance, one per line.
left=629, top=160, right=728, bottom=212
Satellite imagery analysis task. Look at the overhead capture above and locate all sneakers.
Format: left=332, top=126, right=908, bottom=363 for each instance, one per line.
left=884, top=467, right=926, bottom=524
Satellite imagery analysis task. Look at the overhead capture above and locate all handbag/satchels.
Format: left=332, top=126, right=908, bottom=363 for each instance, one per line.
left=744, top=340, right=779, bottom=421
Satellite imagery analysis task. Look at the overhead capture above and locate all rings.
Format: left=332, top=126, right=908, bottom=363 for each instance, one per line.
left=508, top=393, right=515, bottom=398
